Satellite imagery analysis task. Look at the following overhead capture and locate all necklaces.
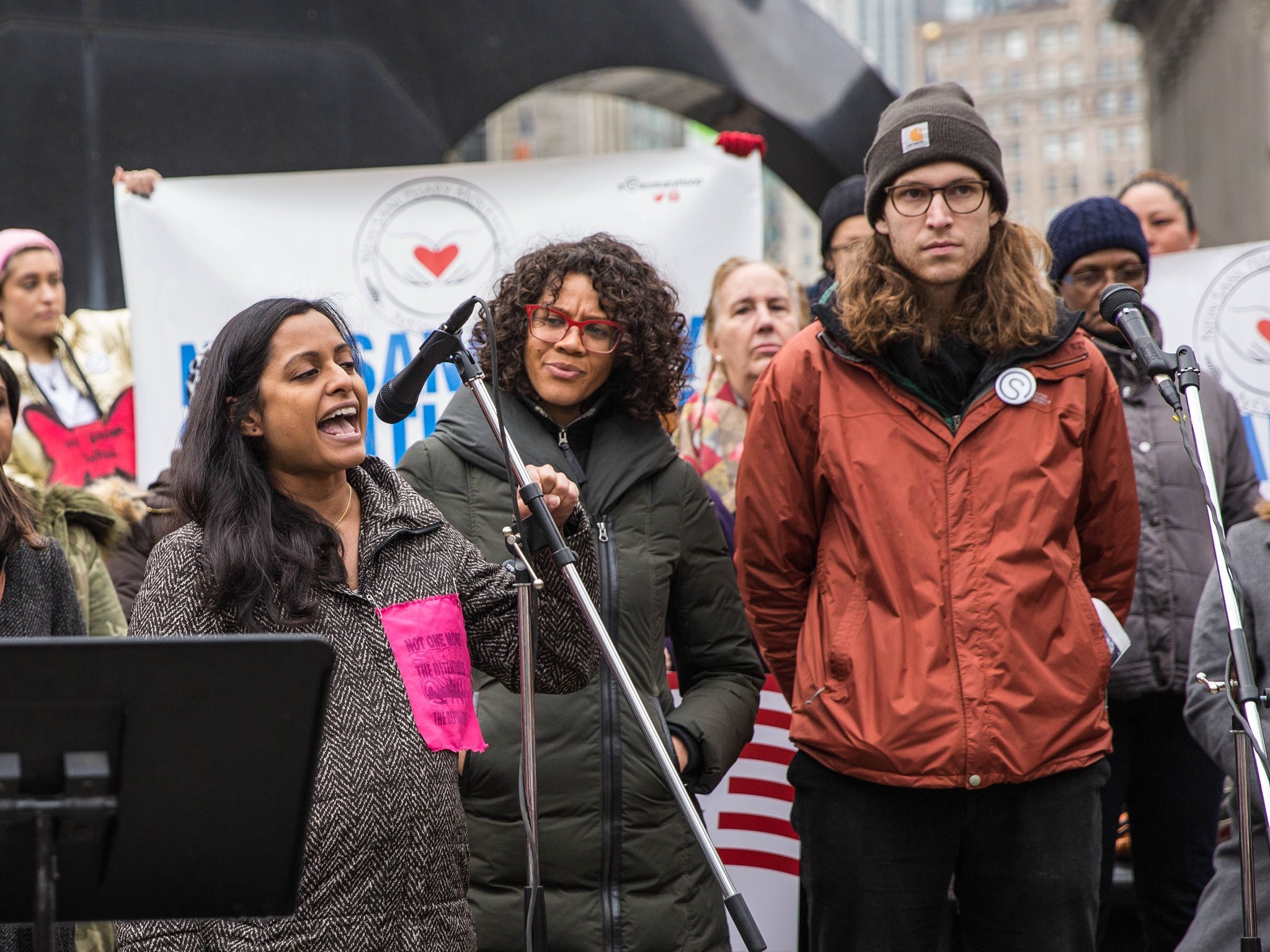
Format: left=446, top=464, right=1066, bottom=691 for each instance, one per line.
left=328, top=482, right=352, bottom=530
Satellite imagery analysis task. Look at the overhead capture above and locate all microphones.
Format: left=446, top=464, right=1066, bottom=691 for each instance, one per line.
left=376, top=297, right=477, bottom=424
left=1099, top=282, right=1182, bottom=410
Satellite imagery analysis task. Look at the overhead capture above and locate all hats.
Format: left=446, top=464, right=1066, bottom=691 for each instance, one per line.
left=0, top=228, right=63, bottom=277
left=1044, top=196, right=1149, bottom=291
left=864, top=82, right=1008, bottom=231
left=822, top=174, right=868, bottom=258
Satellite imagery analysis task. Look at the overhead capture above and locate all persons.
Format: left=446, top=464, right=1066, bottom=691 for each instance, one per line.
left=733, top=79, right=1142, bottom=952
left=0, top=177, right=1270, bottom=952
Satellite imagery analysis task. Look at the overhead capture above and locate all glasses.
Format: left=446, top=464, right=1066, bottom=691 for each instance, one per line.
left=885, top=180, right=989, bottom=217
left=525, top=305, right=627, bottom=355
left=1065, top=263, right=1147, bottom=289
